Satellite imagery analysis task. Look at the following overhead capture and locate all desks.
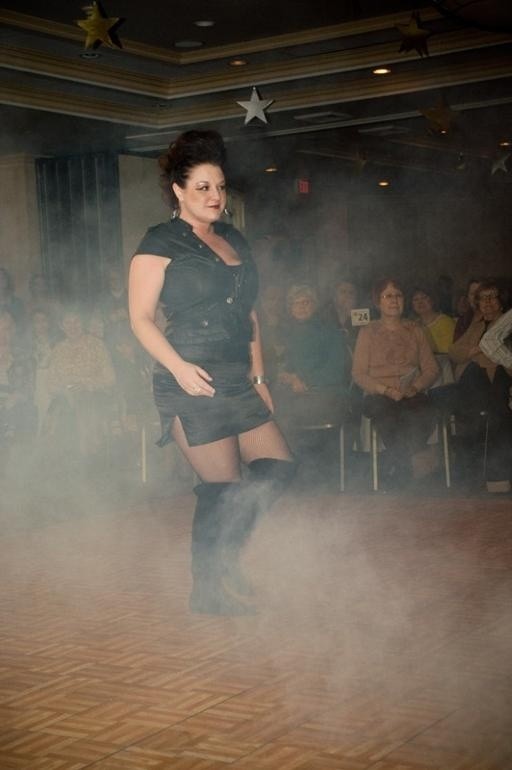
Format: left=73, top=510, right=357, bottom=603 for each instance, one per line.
left=268, top=390, right=363, bottom=495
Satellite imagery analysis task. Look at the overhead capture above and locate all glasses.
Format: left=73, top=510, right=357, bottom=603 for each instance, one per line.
left=377, top=294, right=404, bottom=299
left=294, top=299, right=311, bottom=306
left=479, top=293, right=502, bottom=300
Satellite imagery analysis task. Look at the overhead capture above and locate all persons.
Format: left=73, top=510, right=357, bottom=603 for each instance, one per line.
left=125, top=129, right=298, bottom=618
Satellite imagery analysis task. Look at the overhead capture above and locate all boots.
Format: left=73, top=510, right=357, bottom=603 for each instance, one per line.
left=187, top=456, right=299, bottom=618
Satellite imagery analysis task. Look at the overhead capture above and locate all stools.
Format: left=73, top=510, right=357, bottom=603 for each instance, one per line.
left=366, top=413, right=451, bottom=492
